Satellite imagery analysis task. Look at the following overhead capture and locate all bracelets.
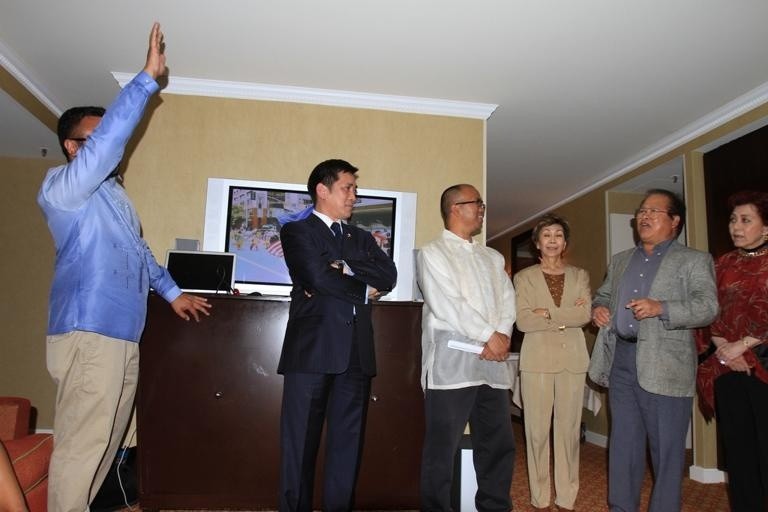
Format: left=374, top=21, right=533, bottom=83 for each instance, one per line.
left=333, top=260, right=345, bottom=274
left=741, top=336, right=750, bottom=350
left=543, top=307, right=551, bottom=319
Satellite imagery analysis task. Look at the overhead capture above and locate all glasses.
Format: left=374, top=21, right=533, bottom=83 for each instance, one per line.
left=635, top=208, right=675, bottom=217
left=452, top=198, right=485, bottom=209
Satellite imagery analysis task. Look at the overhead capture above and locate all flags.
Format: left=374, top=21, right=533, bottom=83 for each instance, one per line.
left=266, top=240, right=284, bottom=259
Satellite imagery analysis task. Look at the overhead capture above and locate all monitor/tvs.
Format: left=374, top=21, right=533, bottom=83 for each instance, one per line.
left=202, top=176, right=418, bottom=301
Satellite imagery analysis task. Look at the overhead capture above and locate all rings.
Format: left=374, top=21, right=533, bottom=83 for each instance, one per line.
left=720, top=359, right=726, bottom=366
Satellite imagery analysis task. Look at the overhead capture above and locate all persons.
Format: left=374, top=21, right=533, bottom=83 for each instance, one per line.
left=411, top=183, right=524, bottom=510
left=513, top=214, right=595, bottom=511
left=36, top=17, right=214, bottom=512
left=274, top=155, right=400, bottom=511
left=586, top=187, right=720, bottom=510
left=250, top=232, right=258, bottom=251
left=0, top=436, right=28, bottom=512
left=690, top=184, right=768, bottom=512
left=235, top=231, right=243, bottom=251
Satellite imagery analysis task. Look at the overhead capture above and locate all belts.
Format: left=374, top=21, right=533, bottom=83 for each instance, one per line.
left=618, top=335, right=638, bottom=343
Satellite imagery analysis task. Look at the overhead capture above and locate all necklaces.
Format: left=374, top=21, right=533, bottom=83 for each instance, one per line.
left=737, top=247, right=768, bottom=260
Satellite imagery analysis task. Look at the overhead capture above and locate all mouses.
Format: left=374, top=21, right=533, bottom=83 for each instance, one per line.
left=248, top=292, right=262, bottom=296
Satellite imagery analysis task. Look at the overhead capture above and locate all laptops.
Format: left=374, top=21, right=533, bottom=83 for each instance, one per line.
left=149, top=249, right=236, bottom=294
